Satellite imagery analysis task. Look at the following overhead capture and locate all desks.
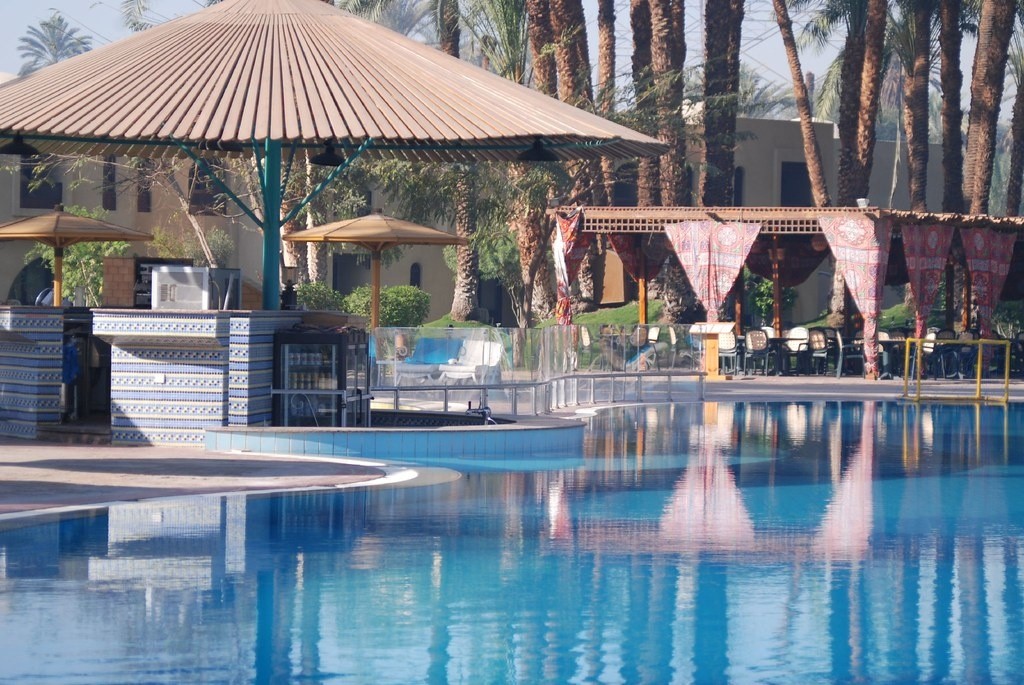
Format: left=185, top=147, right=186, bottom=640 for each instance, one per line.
left=737, top=338, right=907, bottom=380
left=377, top=360, right=401, bottom=388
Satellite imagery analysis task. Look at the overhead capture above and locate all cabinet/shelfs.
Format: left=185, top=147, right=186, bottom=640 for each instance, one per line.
left=271, top=329, right=375, bottom=428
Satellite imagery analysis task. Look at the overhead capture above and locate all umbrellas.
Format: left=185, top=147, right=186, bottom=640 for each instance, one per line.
left=0, top=203, right=156, bottom=306
left=282, top=209, right=470, bottom=330
left=0, top=0, right=670, bottom=311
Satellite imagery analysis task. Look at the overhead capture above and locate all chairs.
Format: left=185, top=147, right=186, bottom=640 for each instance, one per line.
left=394, top=336, right=462, bottom=399
left=437, top=339, right=504, bottom=401
left=577, top=324, right=1024, bottom=381
